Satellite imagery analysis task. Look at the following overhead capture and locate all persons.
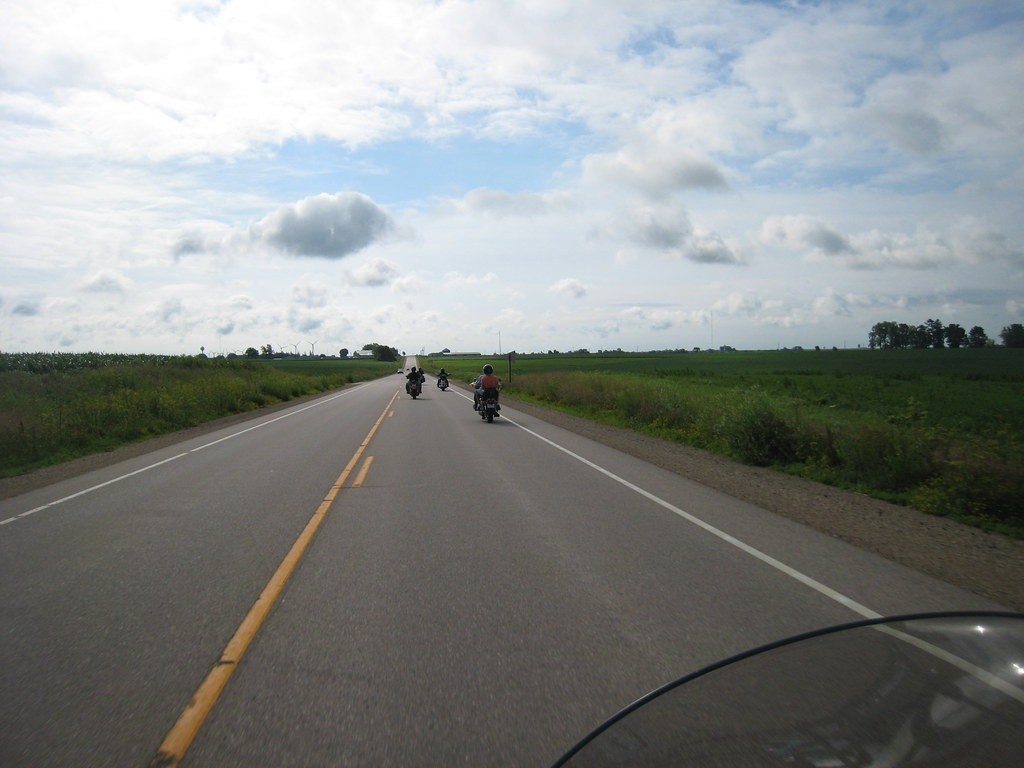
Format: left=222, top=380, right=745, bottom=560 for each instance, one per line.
left=470, top=365, right=500, bottom=417
left=436, top=368, right=450, bottom=387
left=405, top=367, right=425, bottom=394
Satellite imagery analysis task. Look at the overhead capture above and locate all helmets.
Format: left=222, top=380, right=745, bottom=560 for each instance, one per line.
left=441, top=368, right=445, bottom=372
left=411, top=367, right=416, bottom=371
left=419, top=367, right=421, bottom=370
left=483, top=364, right=493, bottom=373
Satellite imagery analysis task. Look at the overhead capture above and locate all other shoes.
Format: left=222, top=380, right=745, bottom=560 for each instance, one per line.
left=473, top=405, right=477, bottom=409
left=496, top=405, right=500, bottom=410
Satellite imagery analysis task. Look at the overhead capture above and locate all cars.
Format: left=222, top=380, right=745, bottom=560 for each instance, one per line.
left=397, top=369, right=404, bottom=374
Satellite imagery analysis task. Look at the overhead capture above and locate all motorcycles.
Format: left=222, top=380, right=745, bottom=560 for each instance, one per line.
left=405, top=375, right=422, bottom=399
left=421, top=374, right=425, bottom=383
left=436, top=373, right=451, bottom=391
left=471, top=377, right=502, bottom=423
